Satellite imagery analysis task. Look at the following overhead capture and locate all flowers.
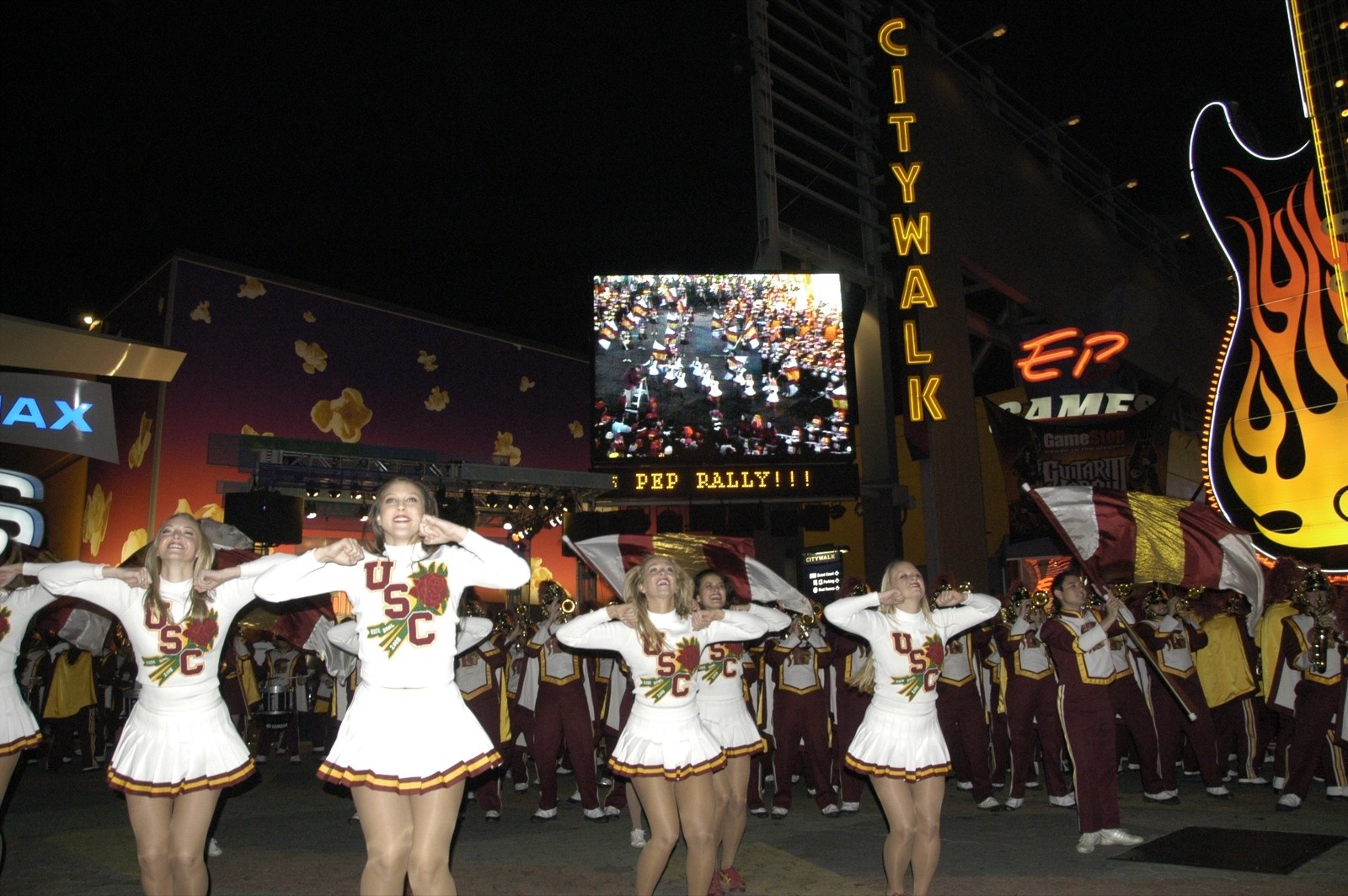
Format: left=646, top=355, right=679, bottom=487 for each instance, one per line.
left=925, top=641, right=944, bottom=665
left=676, top=645, right=699, bottom=673
left=408, top=572, right=447, bottom=609
left=724, top=638, right=744, bottom=654
left=182, top=617, right=218, bottom=648
left=0, top=607, right=12, bottom=642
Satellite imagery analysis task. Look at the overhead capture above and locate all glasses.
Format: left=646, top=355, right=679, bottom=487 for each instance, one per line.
left=1306, top=586, right=1327, bottom=592
left=546, top=599, right=562, bottom=605
left=1149, top=599, right=1167, bottom=605
left=1090, top=601, right=1102, bottom=606
left=1013, top=602, right=1021, bottom=607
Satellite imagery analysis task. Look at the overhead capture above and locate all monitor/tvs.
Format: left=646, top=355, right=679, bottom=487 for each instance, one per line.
left=587, top=265, right=857, bottom=471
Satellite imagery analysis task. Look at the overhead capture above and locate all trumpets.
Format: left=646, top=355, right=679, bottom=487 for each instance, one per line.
left=955, top=580, right=973, bottom=592
left=1086, top=583, right=1133, bottom=604
left=846, top=582, right=867, bottom=597
left=1162, top=583, right=1205, bottom=609
left=1027, top=590, right=1048, bottom=614
left=799, top=603, right=822, bottom=626
left=516, top=604, right=527, bottom=615
left=559, top=598, right=575, bottom=615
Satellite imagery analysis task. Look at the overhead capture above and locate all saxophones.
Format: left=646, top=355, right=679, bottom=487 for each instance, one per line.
left=1314, top=598, right=1327, bottom=673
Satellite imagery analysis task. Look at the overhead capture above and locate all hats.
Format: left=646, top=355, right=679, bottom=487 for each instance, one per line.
left=1298, top=569, right=1334, bottom=611
left=538, top=581, right=567, bottom=618
left=1142, top=586, right=1170, bottom=617
left=1008, top=581, right=1037, bottom=619
left=460, top=597, right=534, bottom=631
left=770, top=579, right=1007, bottom=640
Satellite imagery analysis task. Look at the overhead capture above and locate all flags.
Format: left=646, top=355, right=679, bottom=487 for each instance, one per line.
left=1031, top=486, right=1265, bottom=635
left=574, top=534, right=815, bottom=615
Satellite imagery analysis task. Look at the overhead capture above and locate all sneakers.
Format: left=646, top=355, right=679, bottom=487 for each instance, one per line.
left=1076, top=830, right=1102, bottom=854
left=1099, top=828, right=1143, bottom=847
left=204, top=838, right=222, bottom=857
left=719, top=859, right=746, bottom=892
left=630, top=828, right=646, bottom=848
left=708, top=871, right=726, bottom=896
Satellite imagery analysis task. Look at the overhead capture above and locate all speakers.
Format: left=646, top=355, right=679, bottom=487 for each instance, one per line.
left=434, top=497, right=475, bottom=530
left=223, top=492, right=305, bottom=544
left=561, top=512, right=611, bottom=557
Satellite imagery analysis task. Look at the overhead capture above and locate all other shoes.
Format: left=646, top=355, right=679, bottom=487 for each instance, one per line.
left=19, top=731, right=1347, bottom=824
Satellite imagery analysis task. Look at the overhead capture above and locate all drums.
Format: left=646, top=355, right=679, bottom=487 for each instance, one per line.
left=254, top=686, right=293, bottom=716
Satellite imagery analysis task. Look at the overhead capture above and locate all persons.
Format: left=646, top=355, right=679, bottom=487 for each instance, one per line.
left=15, top=555, right=1348, bottom=849
left=253, top=477, right=530, bottom=896
left=556, top=555, right=792, bottom=896
left=37, top=513, right=300, bottom=896
left=0, top=533, right=68, bottom=808
left=593, top=274, right=852, bottom=462
left=1039, top=570, right=1143, bottom=853
left=824, top=559, right=1002, bottom=896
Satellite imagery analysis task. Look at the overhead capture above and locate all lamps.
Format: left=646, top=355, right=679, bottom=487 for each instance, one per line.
left=304, top=500, right=317, bottom=519
left=486, top=483, right=499, bottom=507
left=304, top=477, right=320, bottom=497
left=357, top=506, right=369, bottom=522
left=544, top=487, right=558, bottom=511
left=327, top=478, right=342, bottom=498
left=350, top=478, right=364, bottom=500
left=562, top=488, right=577, bottom=512
left=371, top=480, right=380, bottom=500
left=507, top=484, right=521, bottom=509
left=502, top=519, right=512, bottom=530
left=527, top=485, right=541, bottom=510
